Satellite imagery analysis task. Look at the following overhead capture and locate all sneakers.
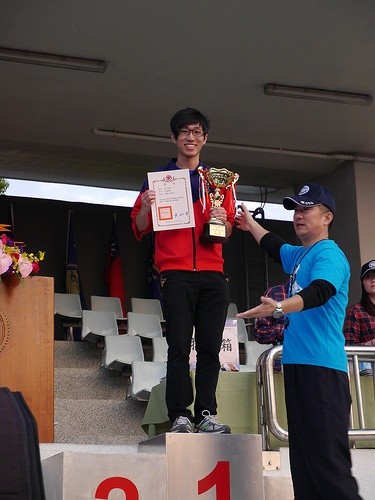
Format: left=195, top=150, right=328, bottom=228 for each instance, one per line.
left=169, top=416, right=193, bottom=433
left=194, top=410, right=231, bottom=434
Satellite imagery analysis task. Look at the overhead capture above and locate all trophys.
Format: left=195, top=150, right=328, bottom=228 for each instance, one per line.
left=198, top=166, right=239, bottom=243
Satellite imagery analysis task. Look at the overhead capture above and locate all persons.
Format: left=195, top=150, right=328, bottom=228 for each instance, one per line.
left=131, top=108, right=239, bottom=433
left=343, top=260, right=375, bottom=370
left=236, top=183, right=364, bottom=500
left=255, top=284, right=289, bottom=372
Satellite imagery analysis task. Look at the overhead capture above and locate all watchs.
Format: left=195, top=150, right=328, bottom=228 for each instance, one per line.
left=273, top=300, right=284, bottom=319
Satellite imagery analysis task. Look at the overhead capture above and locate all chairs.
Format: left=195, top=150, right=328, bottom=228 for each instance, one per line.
left=54, top=292, right=281, bottom=401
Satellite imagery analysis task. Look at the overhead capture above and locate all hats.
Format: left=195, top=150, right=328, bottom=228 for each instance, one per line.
left=360, top=259, right=375, bottom=278
left=282, top=183, right=337, bottom=216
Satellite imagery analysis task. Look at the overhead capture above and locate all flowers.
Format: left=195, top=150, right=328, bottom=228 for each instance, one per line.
left=0, top=222, right=47, bottom=289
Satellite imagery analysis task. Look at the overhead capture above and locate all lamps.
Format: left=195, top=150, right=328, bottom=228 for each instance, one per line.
left=263, top=83, right=372, bottom=106
left=0, top=49, right=107, bottom=73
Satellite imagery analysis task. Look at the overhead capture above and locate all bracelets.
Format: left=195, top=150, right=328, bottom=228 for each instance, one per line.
left=371, top=340, right=373, bottom=347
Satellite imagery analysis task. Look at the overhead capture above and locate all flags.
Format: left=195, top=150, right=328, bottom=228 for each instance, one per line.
left=105, top=212, right=127, bottom=336
left=62, top=211, right=88, bottom=340
left=145, top=231, right=166, bottom=318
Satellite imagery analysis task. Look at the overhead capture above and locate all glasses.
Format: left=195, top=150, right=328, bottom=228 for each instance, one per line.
left=177, top=127, right=207, bottom=137
